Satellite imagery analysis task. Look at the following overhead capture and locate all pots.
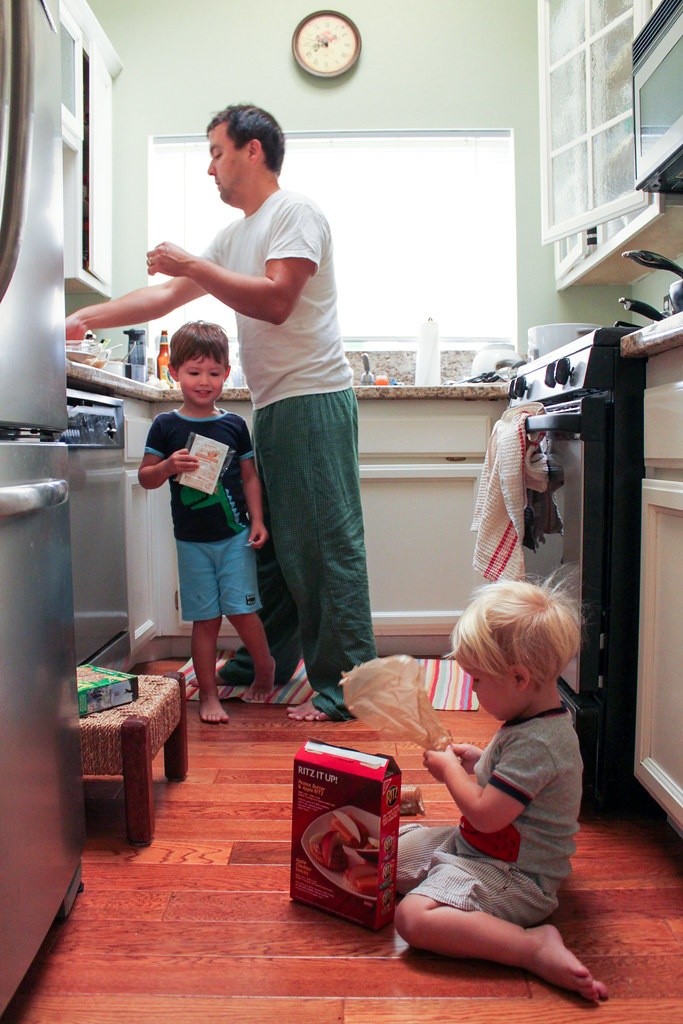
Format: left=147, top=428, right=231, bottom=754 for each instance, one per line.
left=618, top=296, right=672, bottom=323
left=621, top=250, right=683, bottom=314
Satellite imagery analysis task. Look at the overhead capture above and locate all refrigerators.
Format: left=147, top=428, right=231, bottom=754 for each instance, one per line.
left=0, top=0, right=83, bottom=1024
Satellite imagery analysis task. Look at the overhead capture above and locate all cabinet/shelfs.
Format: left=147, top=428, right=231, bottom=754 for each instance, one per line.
left=60, top=0, right=124, bottom=299
left=633, top=349, right=683, bottom=831
left=537, top=0, right=683, bottom=291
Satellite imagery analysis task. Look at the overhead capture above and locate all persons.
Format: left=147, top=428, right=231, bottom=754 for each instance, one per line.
left=66, top=103, right=377, bottom=722
left=396, top=564, right=609, bottom=1001
left=138, top=319, right=276, bottom=722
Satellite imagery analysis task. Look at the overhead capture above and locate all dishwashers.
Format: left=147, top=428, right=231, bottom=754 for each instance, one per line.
left=66, top=388, right=131, bottom=673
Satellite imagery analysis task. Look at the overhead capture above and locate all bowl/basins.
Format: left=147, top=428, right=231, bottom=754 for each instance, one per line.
left=527, top=323, right=604, bottom=362
left=91, top=359, right=107, bottom=368
left=104, top=361, right=123, bottom=376
left=66, top=350, right=97, bottom=363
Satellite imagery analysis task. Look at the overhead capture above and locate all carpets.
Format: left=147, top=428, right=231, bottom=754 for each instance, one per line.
left=176, top=648, right=479, bottom=711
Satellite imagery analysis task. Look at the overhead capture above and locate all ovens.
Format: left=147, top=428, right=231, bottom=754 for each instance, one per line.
left=508, top=394, right=666, bottom=825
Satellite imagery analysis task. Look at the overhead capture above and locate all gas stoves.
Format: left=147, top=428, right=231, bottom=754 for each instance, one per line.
left=508, top=327, right=644, bottom=404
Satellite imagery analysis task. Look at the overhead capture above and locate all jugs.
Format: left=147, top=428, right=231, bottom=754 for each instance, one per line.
left=123, top=328, right=148, bottom=384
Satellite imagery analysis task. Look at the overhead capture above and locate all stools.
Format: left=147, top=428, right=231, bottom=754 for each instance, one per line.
left=79, top=671, right=188, bottom=847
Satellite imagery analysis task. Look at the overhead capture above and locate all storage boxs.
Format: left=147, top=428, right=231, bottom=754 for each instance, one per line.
left=77, top=664, right=138, bottom=716
left=289, top=737, right=402, bottom=932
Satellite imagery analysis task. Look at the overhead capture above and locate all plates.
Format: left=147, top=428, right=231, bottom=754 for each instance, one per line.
left=300, top=805, right=381, bottom=900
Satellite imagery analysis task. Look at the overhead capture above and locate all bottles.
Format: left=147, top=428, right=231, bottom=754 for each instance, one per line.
left=156, top=329, right=174, bottom=388
left=401, top=787, right=424, bottom=814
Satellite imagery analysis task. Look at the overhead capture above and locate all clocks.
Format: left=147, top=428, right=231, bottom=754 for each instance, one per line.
left=291, top=10, right=362, bottom=78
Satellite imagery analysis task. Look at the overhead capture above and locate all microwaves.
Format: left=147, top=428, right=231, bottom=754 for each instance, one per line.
left=632, top=6, right=683, bottom=196
